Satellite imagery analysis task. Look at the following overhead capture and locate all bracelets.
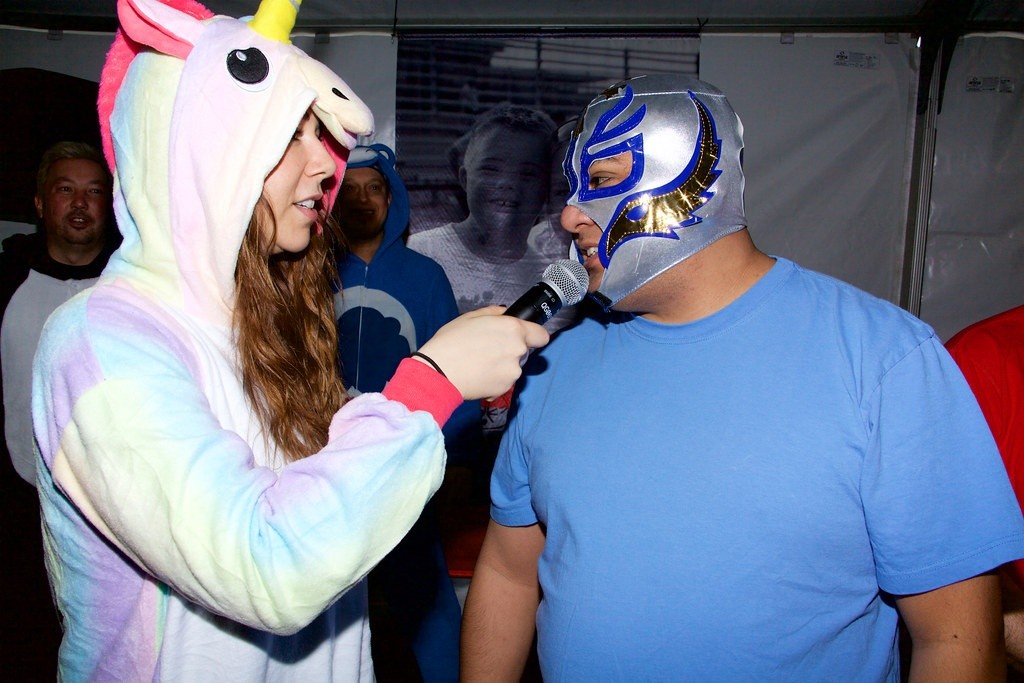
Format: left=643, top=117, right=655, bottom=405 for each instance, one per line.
left=409, top=351, right=448, bottom=381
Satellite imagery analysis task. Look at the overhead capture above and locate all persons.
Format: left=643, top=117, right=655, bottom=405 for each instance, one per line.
left=938, top=304, right=1024, bottom=681
left=400, top=102, right=572, bottom=428
left=0, top=143, right=123, bottom=683
left=456, top=69, right=1024, bottom=683
left=319, top=138, right=484, bottom=683
left=29, top=0, right=555, bottom=683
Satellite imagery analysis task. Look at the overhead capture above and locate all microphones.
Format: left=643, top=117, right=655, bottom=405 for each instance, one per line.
left=501, top=259, right=590, bottom=325
